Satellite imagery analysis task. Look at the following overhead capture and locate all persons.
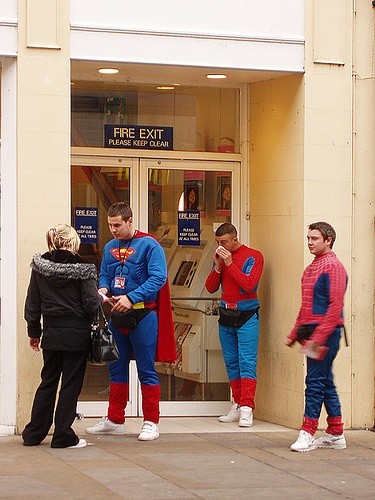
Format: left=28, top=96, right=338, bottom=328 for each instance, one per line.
left=220, top=185, right=231, bottom=209
left=204, top=223, right=264, bottom=427
left=85, top=201, right=177, bottom=441
left=187, top=187, right=198, bottom=211
left=284, top=222, right=349, bottom=452
left=22, top=223, right=103, bottom=449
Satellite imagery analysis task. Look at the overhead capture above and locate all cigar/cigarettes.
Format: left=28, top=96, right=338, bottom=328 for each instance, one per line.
left=32, top=350, right=36, bottom=356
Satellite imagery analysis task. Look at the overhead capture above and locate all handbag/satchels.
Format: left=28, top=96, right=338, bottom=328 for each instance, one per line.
left=109, top=307, right=136, bottom=330
left=87, top=304, right=120, bottom=366
left=215, top=305, right=241, bottom=329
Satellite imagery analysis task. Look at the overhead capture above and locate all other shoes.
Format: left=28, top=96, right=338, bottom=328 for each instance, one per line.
left=67, top=439, right=88, bottom=448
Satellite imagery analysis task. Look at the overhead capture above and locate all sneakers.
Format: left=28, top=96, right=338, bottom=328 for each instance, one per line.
left=239, top=406, right=254, bottom=427
left=137, top=420, right=160, bottom=440
left=290, top=430, right=316, bottom=452
left=313, top=431, right=347, bottom=450
left=218, top=403, right=239, bottom=423
left=85, top=416, right=125, bottom=435
